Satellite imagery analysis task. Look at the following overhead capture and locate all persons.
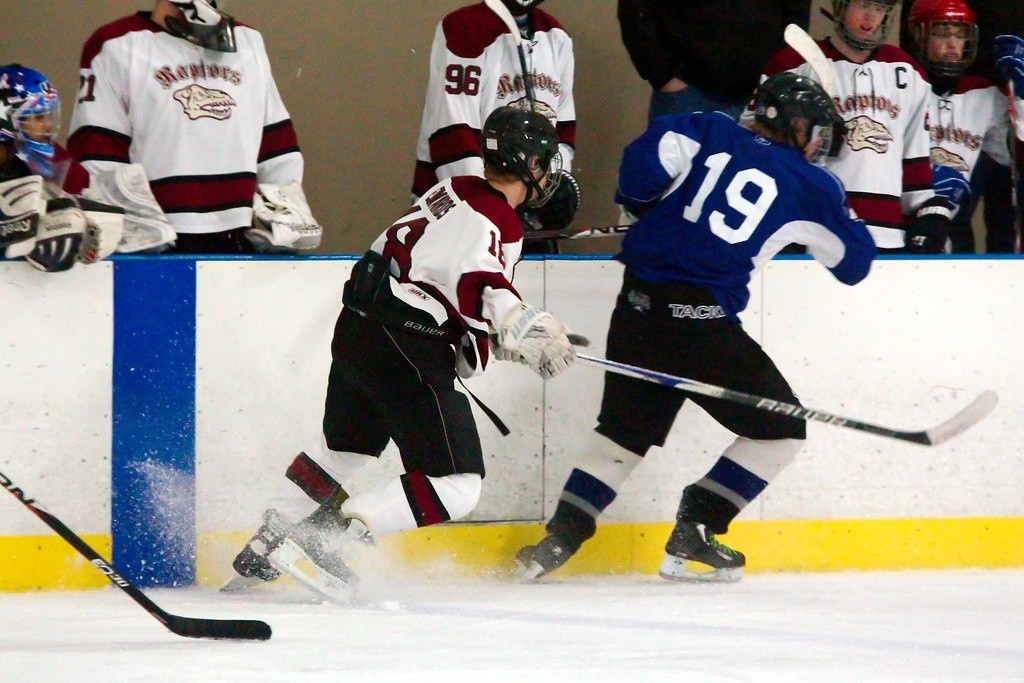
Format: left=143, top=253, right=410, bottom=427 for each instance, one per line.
left=617, top=0, right=811, bottom=127
left=739, top=0, right=1024, bottom=254
left=517, top=73, right=875, bottom=584
left=65, top=0, right=323, bottom=254
left=219, top=107, right=574, bottom=607
left=409, top=0, right=578, bottom=254
left=0, top=63, right=126, bottom=271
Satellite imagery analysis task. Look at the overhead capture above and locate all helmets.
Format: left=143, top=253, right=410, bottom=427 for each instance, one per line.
left=757, top=72, right=846, bottom=132
left=909, top=0, right=977, bottom=77
left=833, top=0, right=897, bottom=49
left=478, top=107, right=561, bottom=167
left=0, top=63, right=60, bottom=178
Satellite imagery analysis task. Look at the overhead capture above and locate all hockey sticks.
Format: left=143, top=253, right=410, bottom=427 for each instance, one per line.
left=1007, top=65, right=1023, bottom=255
left=569, top=350, right=1000, bottom=446
left=784, top=22, right=841, bottom=121
left=0, top=472, right=273, bottom=640
left=485, top=0, right=537, bottom=112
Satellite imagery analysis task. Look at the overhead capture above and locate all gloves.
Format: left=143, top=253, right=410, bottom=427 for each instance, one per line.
left=905, top=198, right=960, bottom=255
left=23, top=199, right=84, bottom=272
left=0, top=174, right=44, bottom=246
left=491, top=305, right=591, bottom=377
left=515, top=170, right=581, bottom=231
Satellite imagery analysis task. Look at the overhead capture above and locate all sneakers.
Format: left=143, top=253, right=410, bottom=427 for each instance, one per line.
left=219, top=510, right=319, bottom=604
left=660, top=522, right=747, bottom=583
left=269, top=504, right=392, bottom=606
left=513, top=538, right=568, bottom=581
